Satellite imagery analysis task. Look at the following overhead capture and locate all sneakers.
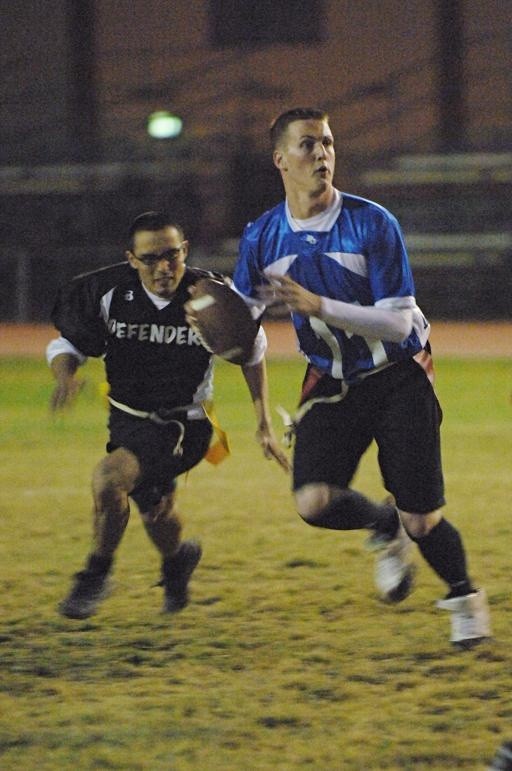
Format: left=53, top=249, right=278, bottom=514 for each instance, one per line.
left=437, top=586, right=494, bottom=649
left=363, top=504, right=417, bottom=607
left=56, top=566, right=113, bottom=620
left=156, top=540, right=204, bottom=618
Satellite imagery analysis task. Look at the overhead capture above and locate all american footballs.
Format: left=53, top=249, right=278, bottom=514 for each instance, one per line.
left=191, top=280, right=254, bottom=366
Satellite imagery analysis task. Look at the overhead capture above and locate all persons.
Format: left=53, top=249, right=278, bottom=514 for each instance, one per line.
left=180, top=104, right=495, bottom=651
left=44, top=210, right=294, bottom=623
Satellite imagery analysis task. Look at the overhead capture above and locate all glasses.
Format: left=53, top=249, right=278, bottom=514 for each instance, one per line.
left=130, top=244, right=183, bottom=266
